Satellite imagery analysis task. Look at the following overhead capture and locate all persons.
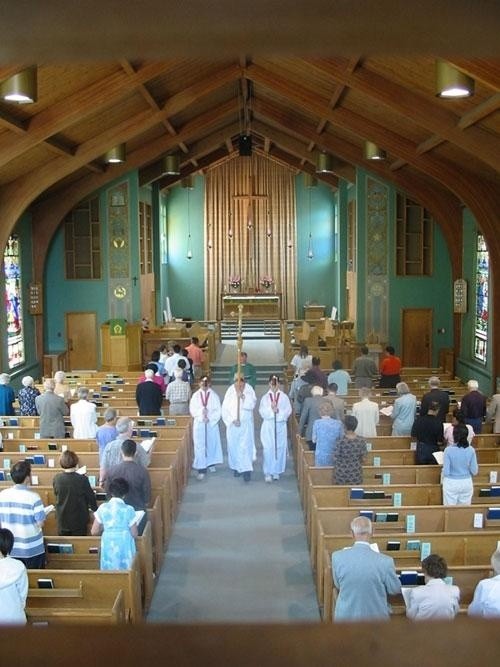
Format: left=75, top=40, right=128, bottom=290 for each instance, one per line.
left=466, top=543, right=500, bottom=619
left=404, top=552, right=463, bottom=621
left=52, top=451, right=97, bottom=537
left=1, top=529, right=30, bottom=625
left=1, top=335, right=205, bottom=438
left=102, top=441, right=153, bottom=535
left=229, top=352, right=258, bottom=386
left=260, top=375, right=292, bottom=483
left=190, top=375, right=222, bottom=481
left=222, top=372, right=259, bottom=481
left=0, top=459, right=49, bottom=568
left=91, top=479, right=140, bottom=575
left=289, top=344, right=500, bottom=506
left=97, top=417, right=158, bottom=491
left=331, top=517, right=402, bottom=621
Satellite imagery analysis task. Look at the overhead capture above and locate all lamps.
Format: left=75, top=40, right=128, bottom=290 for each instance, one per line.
left=304, top=168, right=318, bottom=188
left=182, top=172, right=196, bottom=189
left=165, top=152, right=180, bottom=176
left=318, top=149, right=336, bottom=173
left=1, top=60, right=40, bottom=106
left=103, top=139, right=127, bottom=165
left=365, top=138, right=387, bottom=161
left=436, top=57, right=474, bottom=98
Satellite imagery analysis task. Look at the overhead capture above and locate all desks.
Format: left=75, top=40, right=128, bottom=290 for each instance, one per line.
left=222, top=293, right=283, bottom=321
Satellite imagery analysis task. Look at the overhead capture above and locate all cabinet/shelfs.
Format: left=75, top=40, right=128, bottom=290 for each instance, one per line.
left=41, top=348, right=70, bottom=377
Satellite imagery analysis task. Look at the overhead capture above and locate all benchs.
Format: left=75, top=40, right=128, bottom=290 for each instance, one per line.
left=276, top=359, right=498, bottom=619
left=0, top=364, right=200, bottom=623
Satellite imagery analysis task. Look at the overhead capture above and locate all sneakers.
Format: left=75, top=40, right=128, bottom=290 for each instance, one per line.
left=196, top=466, right=280, bottom=483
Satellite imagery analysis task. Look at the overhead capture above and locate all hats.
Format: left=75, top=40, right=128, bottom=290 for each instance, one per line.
left=234, top=372, right=244, bottom=381
